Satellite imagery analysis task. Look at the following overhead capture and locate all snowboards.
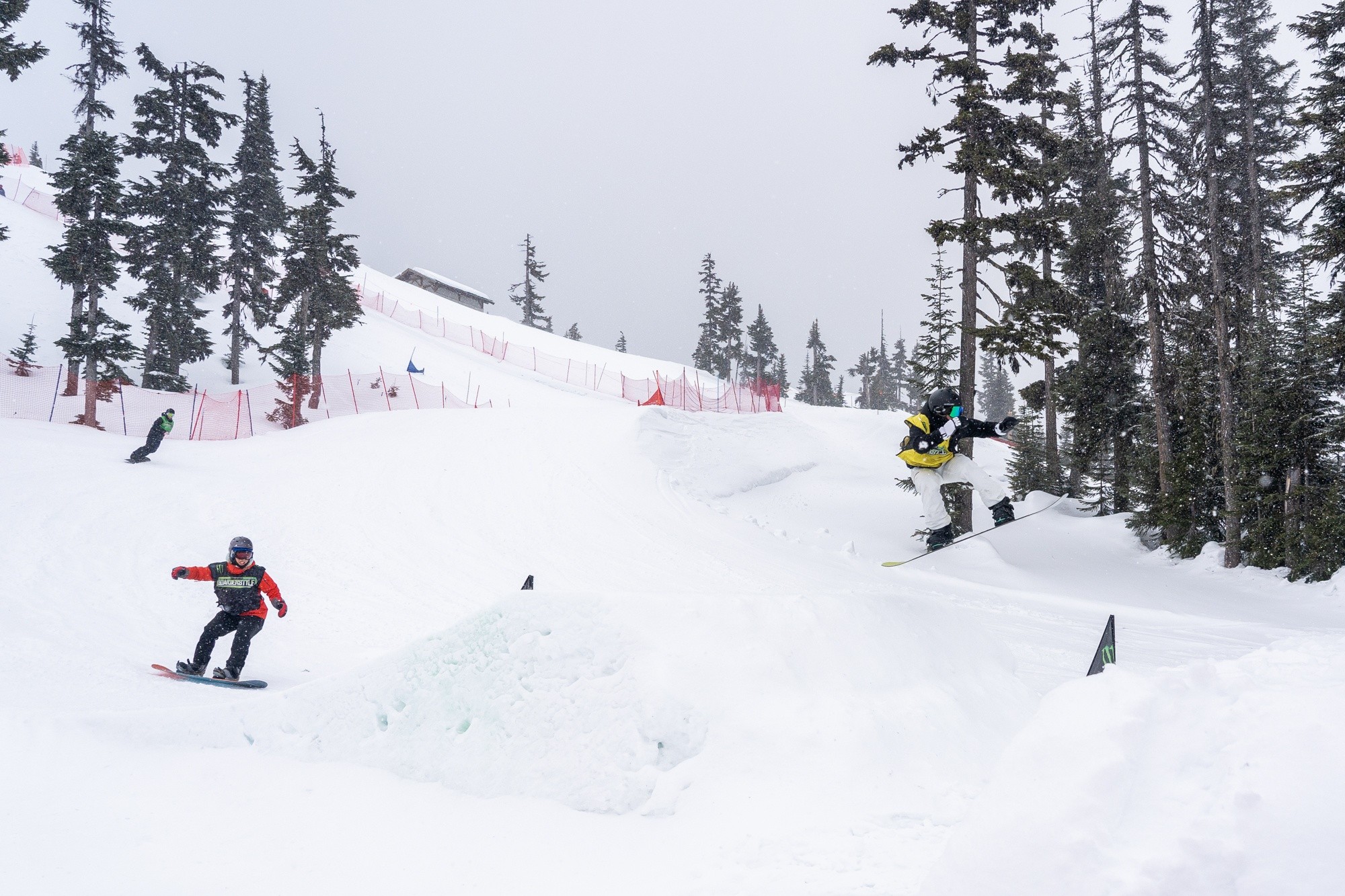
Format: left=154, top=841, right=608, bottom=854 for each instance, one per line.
left=123, top=451, right=151, bottom=464
left=151, top=660, right=268, bottom=689
left=881, top=491, right=1069, bottom=567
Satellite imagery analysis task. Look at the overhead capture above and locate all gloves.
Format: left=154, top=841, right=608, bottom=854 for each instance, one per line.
left=171, top=567, right=187, bottom=581
left=999, top=417, right=1022, bottom=433
left=942, top=415, right=968, bottom=436
left=277, top=601, right=288, bottom=618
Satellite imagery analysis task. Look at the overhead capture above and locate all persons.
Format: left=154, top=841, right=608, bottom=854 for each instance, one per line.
left=895, top=389, right=1023, bottom=551
left=0, top=185, right=5, bottom=197
left=130, top=407, right=175, bottom=462
left=171, top=537, right=289, bottom=683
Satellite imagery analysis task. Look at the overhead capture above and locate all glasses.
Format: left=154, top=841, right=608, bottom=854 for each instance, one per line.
left=943, top=407, right=964, bottom=417
left=234, top=551, right=253, bottom=559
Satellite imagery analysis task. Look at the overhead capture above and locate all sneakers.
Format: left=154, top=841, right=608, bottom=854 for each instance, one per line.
left=176, top=661, right=206, bottom=675
left=929, top=529, right=953, bottom=549
left=213, top=665, right=241, bottom=680
left=991, top=505, right=1016, bottom=527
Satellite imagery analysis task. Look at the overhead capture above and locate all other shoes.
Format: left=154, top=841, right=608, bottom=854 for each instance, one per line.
left=131, top=456, right=146, bottom=462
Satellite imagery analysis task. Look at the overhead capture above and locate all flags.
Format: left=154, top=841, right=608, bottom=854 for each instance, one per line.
left=637, top=389, right=665, bottom=406
left=406, top=360, right=424, bottom=374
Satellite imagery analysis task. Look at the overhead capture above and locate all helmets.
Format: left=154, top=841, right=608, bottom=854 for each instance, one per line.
left=229, top=536, right=253, bottom=550
left=929, top=388, right=962, bottom=412
left=166, top=408, right=175, bottom=414
